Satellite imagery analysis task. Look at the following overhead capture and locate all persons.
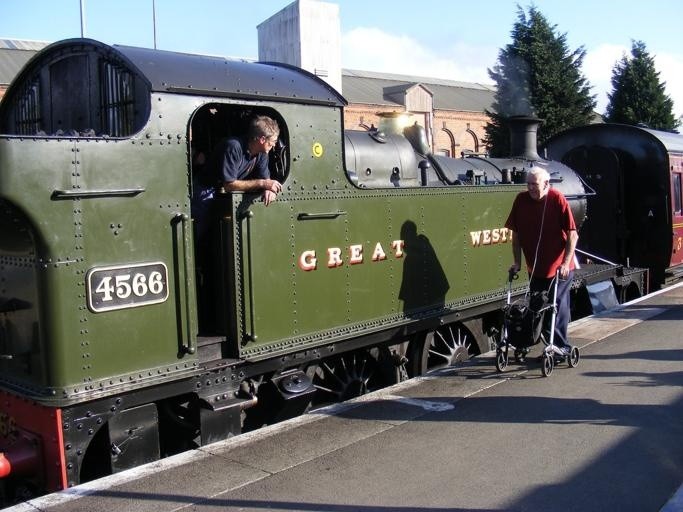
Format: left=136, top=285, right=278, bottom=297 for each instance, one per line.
left=194, top=114, right=283, bottom=207
left=504, top=167, right=580, bottom=363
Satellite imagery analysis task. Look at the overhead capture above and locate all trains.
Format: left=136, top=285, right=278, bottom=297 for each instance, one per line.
left=1, top=38, right=683, bottom=505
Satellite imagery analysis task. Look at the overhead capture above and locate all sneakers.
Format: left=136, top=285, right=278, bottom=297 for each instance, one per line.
left=554, top=354, right=566, bottom=363
